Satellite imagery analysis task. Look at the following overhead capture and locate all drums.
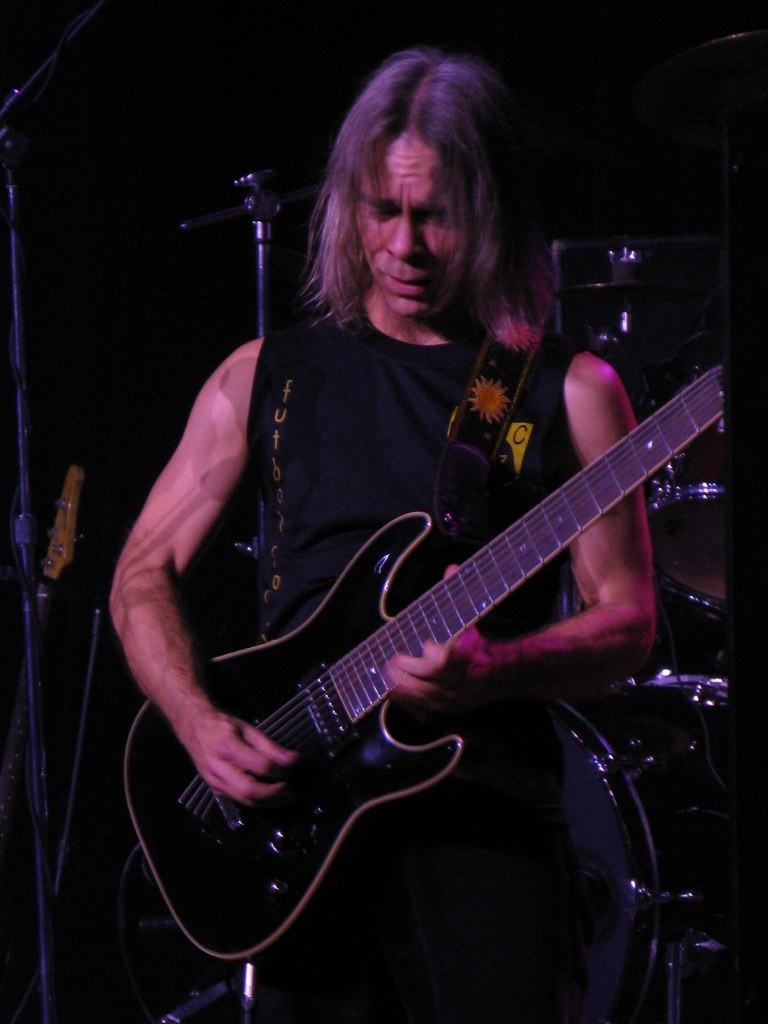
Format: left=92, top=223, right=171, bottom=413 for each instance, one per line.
left=570, top=481, right=727, bottom=674
left=407, top=673, right=735, bottom=1024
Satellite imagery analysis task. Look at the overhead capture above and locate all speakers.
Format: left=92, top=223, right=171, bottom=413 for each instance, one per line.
left=713, top=101, right=768, bottom=1024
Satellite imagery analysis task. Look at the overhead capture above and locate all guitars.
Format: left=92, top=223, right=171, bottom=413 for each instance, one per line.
left=0, top=464, right=87, bottom=854
left=123, top=364, right=725, bottom=961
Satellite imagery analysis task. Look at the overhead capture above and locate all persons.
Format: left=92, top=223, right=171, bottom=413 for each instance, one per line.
left=109, top=44, right=655, bottom=1024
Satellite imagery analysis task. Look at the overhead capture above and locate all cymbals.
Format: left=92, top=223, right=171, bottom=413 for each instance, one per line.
left=633, top=25, right=768, bottom=127
left=551, top=281, right=679, bottom=303
